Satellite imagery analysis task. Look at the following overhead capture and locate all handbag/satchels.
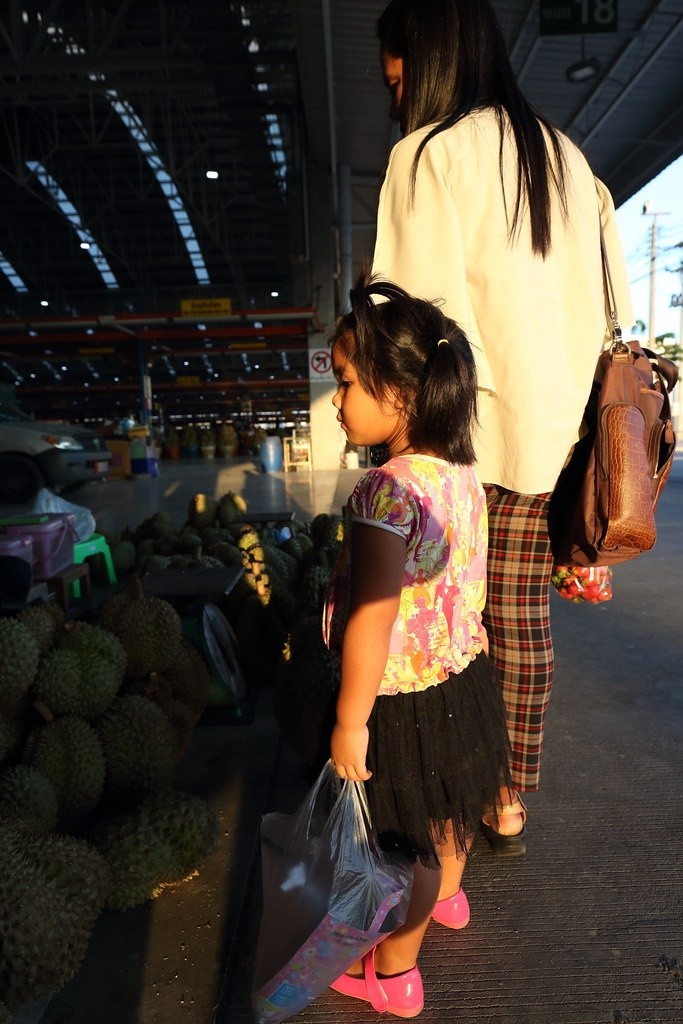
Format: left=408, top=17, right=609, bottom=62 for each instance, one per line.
left=32, top=489, right=96, bottom=544
left=251, top=760, right=412, bottom=1024
left=556, top=340, right=679, bottom=567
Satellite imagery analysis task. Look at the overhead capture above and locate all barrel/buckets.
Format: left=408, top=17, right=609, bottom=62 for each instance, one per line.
left=261, top=437, right=282, bottom=472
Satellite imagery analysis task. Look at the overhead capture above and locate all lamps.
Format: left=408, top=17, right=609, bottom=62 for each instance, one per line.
left=564, top=35, right=601, bottom=80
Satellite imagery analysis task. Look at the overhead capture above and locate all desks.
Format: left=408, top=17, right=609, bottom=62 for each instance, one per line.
left=121, top=566, right=246, bottom=618
left=229, top=512, right=295, bottom=529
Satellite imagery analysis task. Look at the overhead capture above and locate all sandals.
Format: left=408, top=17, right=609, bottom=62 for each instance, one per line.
left=480, top=792, right=528, bottom=857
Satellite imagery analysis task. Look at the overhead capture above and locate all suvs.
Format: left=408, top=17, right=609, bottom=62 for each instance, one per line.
left=1, top=401, right=115, bottom=504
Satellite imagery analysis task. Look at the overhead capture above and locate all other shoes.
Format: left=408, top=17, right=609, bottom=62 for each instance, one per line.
left=330, top=946, right=424, bottom=1018
left=431, top=888, right=469, bottom=930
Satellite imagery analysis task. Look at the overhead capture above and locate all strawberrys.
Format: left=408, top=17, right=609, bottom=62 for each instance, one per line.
left=550, top=566, right=612, bottom=605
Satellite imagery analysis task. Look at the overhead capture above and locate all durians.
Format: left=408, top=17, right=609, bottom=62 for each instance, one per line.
left=0, top=575, right=218, bottom=1024
left=107, top=492, right=344, bottom=622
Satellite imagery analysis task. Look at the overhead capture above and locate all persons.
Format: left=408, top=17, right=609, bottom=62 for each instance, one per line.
left=322, top=296, right=514, bottom=1018
left=370, top=2, right=630, bottom=862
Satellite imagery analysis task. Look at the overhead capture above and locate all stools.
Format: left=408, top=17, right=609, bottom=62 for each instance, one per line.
left=47, top=563, right=91, bottom=611
left=7, top=513, right=78, bottom=578
left=60, top=532, right=118, bottom=601
left=0, top=534, right=35, bottom=586
